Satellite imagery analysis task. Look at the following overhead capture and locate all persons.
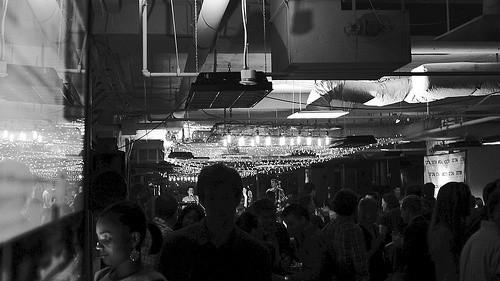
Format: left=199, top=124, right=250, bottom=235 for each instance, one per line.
left=1, top=157, right=83, bottom=281
left=91, top=164, right=500, bottom=281
